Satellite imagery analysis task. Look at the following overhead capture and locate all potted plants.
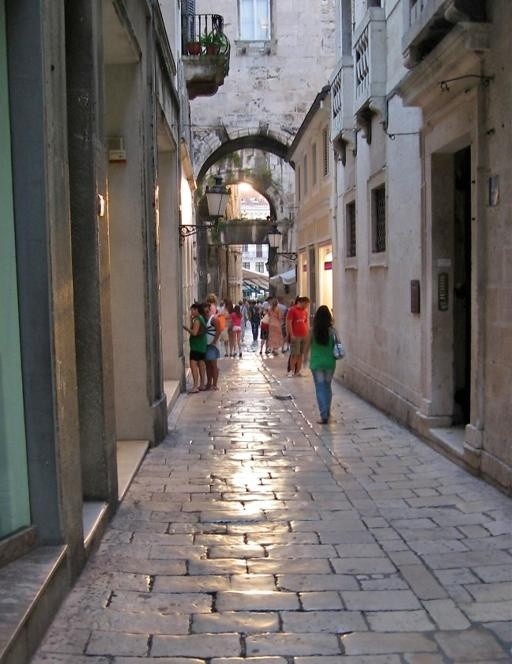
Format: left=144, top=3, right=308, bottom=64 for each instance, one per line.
left=198, top=30, right=227, bottom=55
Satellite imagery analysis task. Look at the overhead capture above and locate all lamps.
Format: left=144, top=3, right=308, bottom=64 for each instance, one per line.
left=267, top=224, right=298, bottom=266
left=178, top=174, right=230, bottom=246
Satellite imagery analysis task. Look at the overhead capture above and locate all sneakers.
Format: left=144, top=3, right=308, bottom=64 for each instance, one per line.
left=285, top=369, right=306, bottom=377
left=188, top=385, right=218, bottom=393
left=317, top=415, right=329, bottom=424
left=224, top=352, right=243, bottom=357
left=259, top=351, right=281, bottom=356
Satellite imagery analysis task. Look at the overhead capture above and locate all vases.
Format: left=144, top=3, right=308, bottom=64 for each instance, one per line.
left=186, top=42, right=201, bottom=55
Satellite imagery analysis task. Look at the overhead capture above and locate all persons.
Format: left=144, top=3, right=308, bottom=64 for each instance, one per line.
left=181, top=291, right=310, bottom=392
left=303, top=305, right=341, bottom=424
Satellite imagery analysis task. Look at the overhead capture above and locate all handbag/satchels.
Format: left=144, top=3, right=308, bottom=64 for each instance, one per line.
left=331, top=327, right=345, bottom=359
